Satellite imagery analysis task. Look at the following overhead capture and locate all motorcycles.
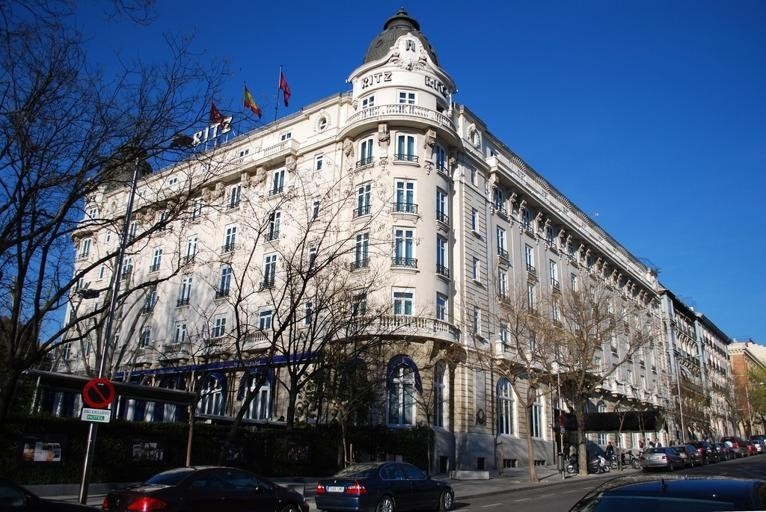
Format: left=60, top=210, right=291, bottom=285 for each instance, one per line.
left=567, top=451, right=640, bottom=474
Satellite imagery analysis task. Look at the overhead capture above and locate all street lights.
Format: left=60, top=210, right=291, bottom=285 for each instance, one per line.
left=675, top=355, right=703, bottom=443
left=744, top=383, right=764, bottom=435
left=77, top=135, right=196, bottom=501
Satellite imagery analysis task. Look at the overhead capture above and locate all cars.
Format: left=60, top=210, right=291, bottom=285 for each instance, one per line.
left=567, top=472, right=766, bottom=511
left=102, top=465, right=308, bottom=512
left=1, top=478, right=102, bottom=511
left=639, top=435, right=765, bottom=471
left=315, top=462, right=454, bottom=512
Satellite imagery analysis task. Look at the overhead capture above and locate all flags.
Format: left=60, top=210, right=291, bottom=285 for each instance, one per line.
left=244, top=85, right=262, bottom=119
left=209, top=102, right=229, bottom=130
left=279, top=71, right=291, bottom=107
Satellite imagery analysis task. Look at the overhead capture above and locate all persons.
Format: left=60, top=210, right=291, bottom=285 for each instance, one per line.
left=637, top=438, right=661, bottom=457
left=563, top=442, right=576, bottom=463
left=605, top=442, right=614, bottom=461
